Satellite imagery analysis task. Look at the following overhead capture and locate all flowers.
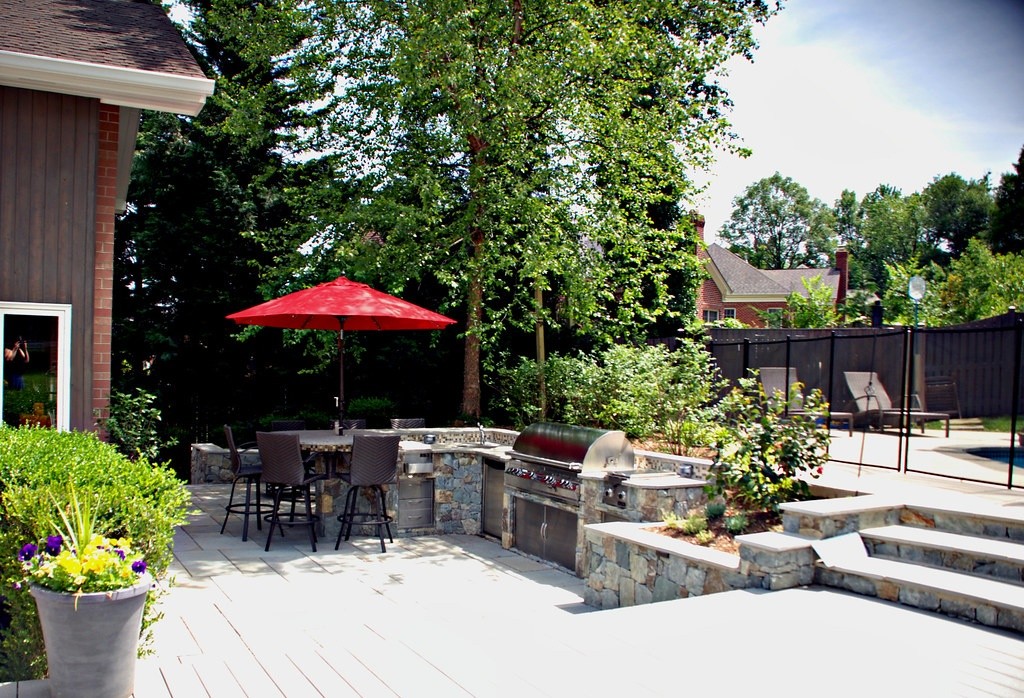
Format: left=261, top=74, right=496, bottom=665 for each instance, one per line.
left=9, top=477, right=147, bottom=613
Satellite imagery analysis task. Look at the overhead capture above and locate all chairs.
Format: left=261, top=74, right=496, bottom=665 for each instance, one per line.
left=844, top=371, right=950, bottom=437
left=759, top=367, right=854, bottom=437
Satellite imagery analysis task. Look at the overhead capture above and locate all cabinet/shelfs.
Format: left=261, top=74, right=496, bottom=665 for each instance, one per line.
left=513, top=497, right=578, bottom=572
left=398, top=452, right=435, bottom=529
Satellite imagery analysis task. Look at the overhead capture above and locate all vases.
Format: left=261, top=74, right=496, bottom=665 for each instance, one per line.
left=30, top=572, right=153, bottom=698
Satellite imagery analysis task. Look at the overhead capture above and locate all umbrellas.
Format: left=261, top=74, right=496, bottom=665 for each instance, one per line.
left=225, top=276, right=457, bottom=435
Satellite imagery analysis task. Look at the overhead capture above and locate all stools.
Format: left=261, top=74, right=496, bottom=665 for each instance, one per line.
left=335, top=420, right=366, bottom=429
left=390, top=419, right=425, bottom=429
left=335, top=435, right=400, bottom=553
left=220, top=420, right=324, bottom=552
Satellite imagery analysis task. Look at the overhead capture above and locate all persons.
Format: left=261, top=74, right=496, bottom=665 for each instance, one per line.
left=5, top=334, right=30, bottom=391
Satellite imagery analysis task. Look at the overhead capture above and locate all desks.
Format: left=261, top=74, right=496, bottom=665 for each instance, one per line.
left=275, top=430, right=402, bottom=536
